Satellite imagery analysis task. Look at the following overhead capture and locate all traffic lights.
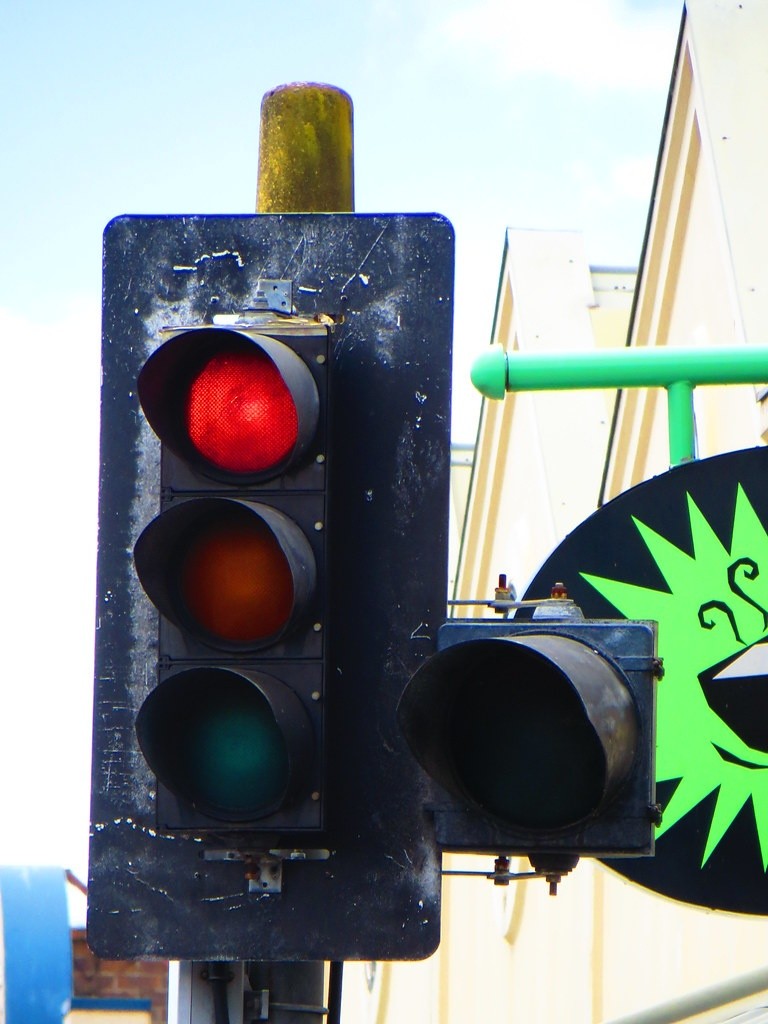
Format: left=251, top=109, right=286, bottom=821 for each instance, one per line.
left=85, top=213, right=455, bottom=964
left=397, top=619, right=660, bottom=858
